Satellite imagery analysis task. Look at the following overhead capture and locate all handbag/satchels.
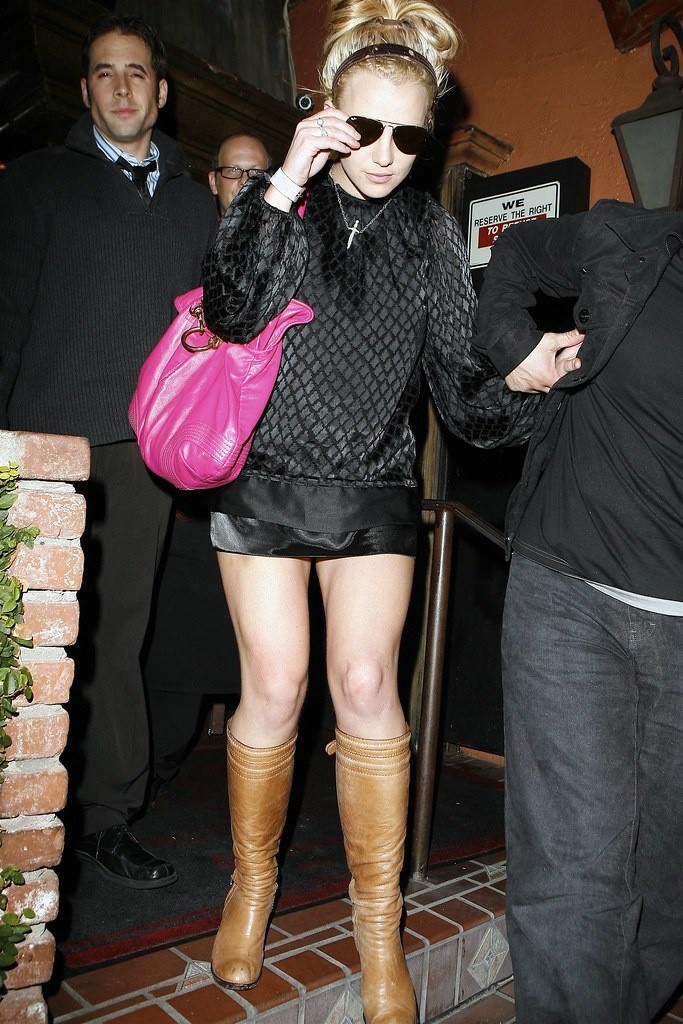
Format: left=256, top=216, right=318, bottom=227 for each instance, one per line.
left=128, top=191, right=314, bottom=492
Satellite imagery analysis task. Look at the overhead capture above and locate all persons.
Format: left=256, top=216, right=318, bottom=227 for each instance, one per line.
left=0, top=15, right=215, bottom=888
left=475, top=197, right=683, bottom=1024
left=137, top=132, right=275, bottom=812
left=205, top=1, right=583, bottom=1024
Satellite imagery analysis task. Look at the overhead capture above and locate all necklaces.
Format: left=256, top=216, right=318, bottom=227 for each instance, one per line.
left=331, top=159, right=393, bottom=249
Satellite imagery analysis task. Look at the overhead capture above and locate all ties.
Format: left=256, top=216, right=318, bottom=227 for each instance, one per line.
left=118, top=156, right=157, bottom=210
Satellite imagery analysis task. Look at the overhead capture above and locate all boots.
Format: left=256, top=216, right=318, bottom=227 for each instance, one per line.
left=325, top=727, right=422, bottom=1024
left=210, top=717, right=298, bottom=992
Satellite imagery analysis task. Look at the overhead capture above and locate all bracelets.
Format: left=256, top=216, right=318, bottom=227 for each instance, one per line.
left=269, top=167, right=306, bottom=203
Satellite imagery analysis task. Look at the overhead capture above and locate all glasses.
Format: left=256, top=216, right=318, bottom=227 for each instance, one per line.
left=213, top=164, right=274, bottom=179
left=345, top=116, right=429, bottom=156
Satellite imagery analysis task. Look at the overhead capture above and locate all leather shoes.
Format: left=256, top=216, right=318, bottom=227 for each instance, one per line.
left=75, top=820, right=177, bottom=889
